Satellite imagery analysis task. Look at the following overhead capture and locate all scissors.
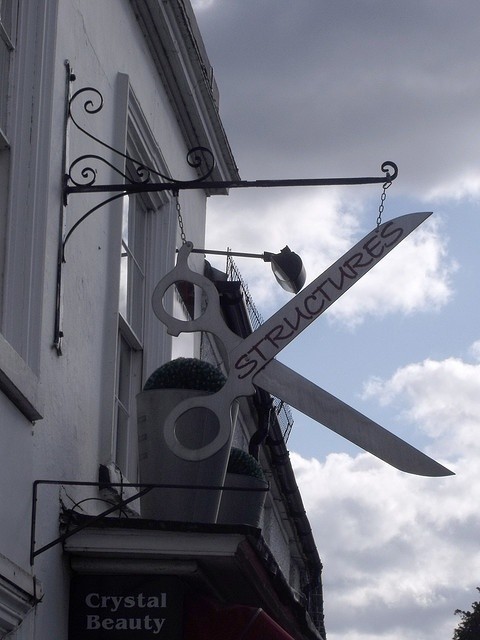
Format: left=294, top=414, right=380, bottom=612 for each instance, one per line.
left=151, top=212, right=456, bottom=477
